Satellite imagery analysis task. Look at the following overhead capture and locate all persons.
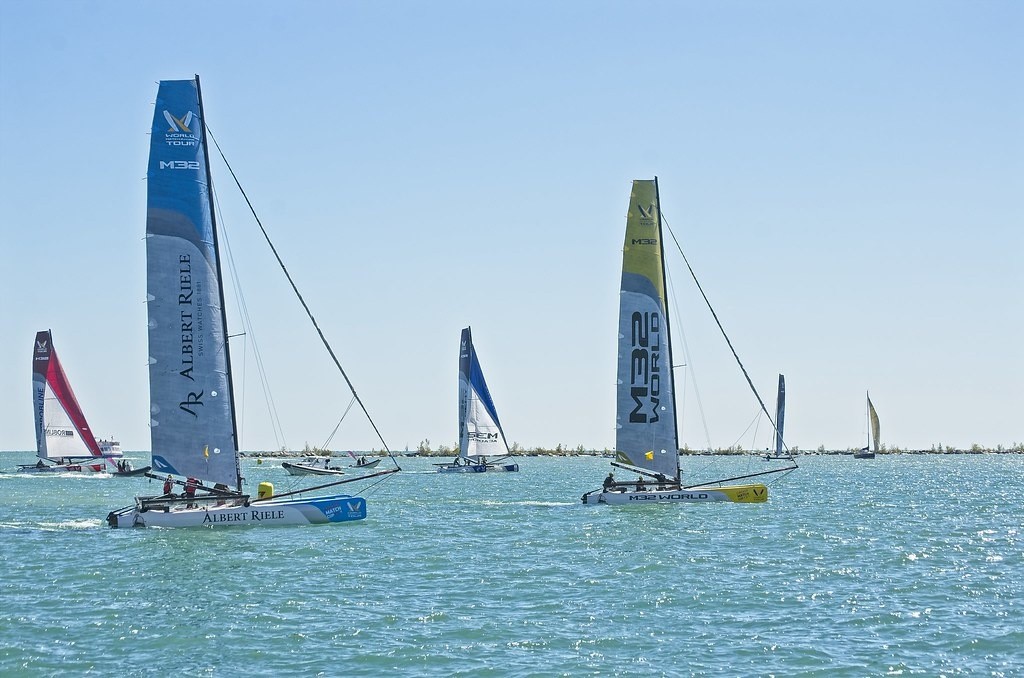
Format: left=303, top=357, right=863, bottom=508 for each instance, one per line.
left=480, top=457, right=487, bottom=465
left=183, top=477, right=203, bottom=508
left=211, top=483, right=230, bottom=506
left=357, top=456, right=367, bottom=465
left=603, top=472, right=618, bottom=492
left=635, top=476, right=646, bottom=491
left=161, top=475, right=174, bottom=513
left=117, top=459, right=130, bottom=472
left=657, top=473, right=668, bottom=491
left=453, top=456, right=461, bottom=467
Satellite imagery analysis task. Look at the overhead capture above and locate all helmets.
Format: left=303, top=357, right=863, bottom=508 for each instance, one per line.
left=168, top=475, right=173, bottom=478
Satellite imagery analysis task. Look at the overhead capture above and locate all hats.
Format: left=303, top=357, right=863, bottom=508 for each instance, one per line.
left=609, top=472, right=613, bottom=475
left=639, top=476, right=643, bottom=479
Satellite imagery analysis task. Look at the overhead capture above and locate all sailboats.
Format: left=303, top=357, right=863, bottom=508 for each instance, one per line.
left=104, top=74, right=403, bottom=529
left=581, top=177, right=798, bottom=504
left=759, top=373, right=796, bottom=460
left=432, top=326, right=520, bottom=473
left=854, top=389, right=880, bottom=459
left=16, top=328, right=108, bottom=475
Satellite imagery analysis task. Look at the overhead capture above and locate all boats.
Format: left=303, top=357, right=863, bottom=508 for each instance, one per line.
left=95, top=436, right=124, bottom=458
left=106, top=458, right=152, bottom=477
left=348, top=451, right=382, bottom=469
left=282, top=452, right=348, bottom=475
